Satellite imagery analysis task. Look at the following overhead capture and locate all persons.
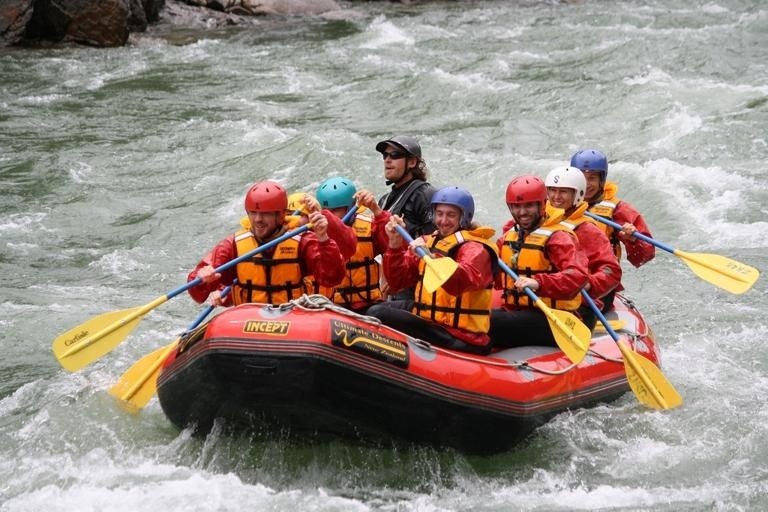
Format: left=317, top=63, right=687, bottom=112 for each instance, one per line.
left=571, top=151, right=656, bottom=314
left=367, top=187, right=498, bottom=357
left=211, top=191, right=356, bottom=308
left=545, top=166, right=622, bottom=334
left=375, top=134, right=437, bottom=239
left=187, top=181, right=345, bottom=309
left=489, top=177, right=587, bottom=352
left=315, top=178, right=408, bottom=313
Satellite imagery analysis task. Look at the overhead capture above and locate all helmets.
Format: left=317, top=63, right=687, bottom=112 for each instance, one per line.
left=285, top=191, right=322, bottom=221
left=375, top=134, right=424, bottom=186
left=570, top=148, right=609, bottom=204
left=244, top=181, right=289, bottom=245
left=430, top=186, right=475, bottom=240
left=505, top=175, right=550, bottom=234
left=315, top=177, right=357, bottom=211
left=544, top=164, right=587, bottom=218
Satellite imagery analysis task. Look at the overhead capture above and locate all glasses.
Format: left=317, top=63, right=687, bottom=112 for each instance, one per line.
left=379, top=149, right=410, bottom=160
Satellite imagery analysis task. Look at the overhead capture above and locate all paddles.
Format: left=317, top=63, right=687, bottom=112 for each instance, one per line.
left=390, top=218, right=459, bottom=294
left=579, top=290, right=683, bottom=411
left=593, top=319, right=627, bottom=333
left=108, top=203, right=308, bottom=410
left=495, top=255, right=593, bottom=366
left=51, top=222, right=317, bottom=373
left=580, top=209, right=760, bottom=295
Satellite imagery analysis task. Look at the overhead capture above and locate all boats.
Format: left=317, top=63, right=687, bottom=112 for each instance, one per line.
left=157, top=295, right=661, bottom=431
